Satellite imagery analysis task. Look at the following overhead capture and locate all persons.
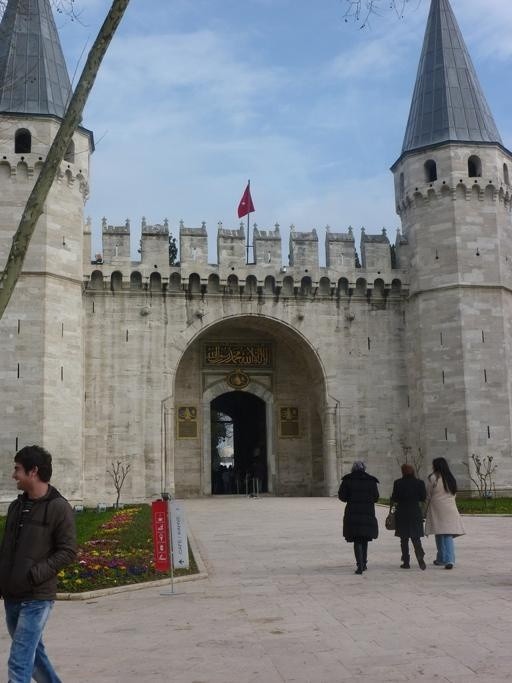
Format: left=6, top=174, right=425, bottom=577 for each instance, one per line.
left=390, top=462, right=426, bottom=571
left=337, top=459, right=380, bottom=574
left=1, top=445, right=76, bottom=682
left=420, top=455, right=465, bottom=569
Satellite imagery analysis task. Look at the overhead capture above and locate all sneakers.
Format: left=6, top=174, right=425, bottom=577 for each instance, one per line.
left=400, top=559, right=426, bottom=571
left=355, top=565, right=368, bottom=574
left=434, top=560, right=453, bottom=569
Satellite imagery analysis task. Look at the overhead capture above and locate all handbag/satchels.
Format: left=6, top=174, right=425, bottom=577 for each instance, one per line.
left=385, top=497, right=398, bottom=531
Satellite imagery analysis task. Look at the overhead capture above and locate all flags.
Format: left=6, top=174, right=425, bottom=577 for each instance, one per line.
left=237, top=184, right=256, bottom=218
